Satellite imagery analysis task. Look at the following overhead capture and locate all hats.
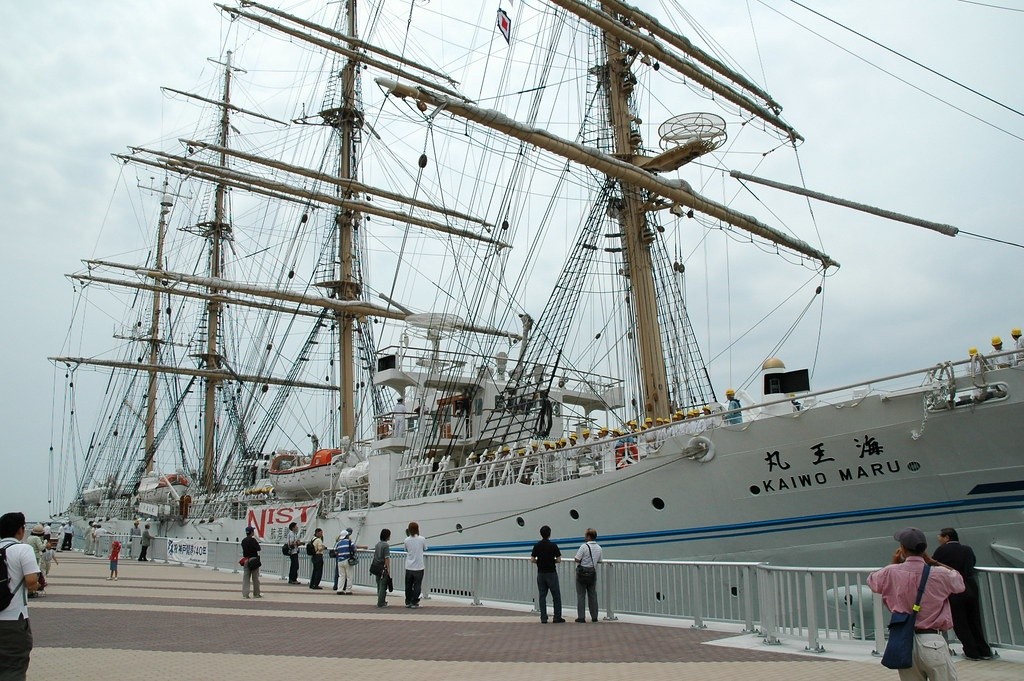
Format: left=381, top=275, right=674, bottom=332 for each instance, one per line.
left=339, top=530, right=348, bottom=539
left=246, top=527, right=255, bottom=533
left=894, top=527, right=927, bottom=551
left=32, top=525, right=43, bottom=534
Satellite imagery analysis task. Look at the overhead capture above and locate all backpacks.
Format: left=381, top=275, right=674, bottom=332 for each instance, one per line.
left=307, top=538, right=318, bottom=555
left=0, top=541, right=27, bottom=611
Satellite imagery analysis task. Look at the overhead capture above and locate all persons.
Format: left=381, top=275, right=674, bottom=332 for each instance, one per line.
left=0, top=512, right=47, bottom=681
left=965, top=329, right=1024, bottom=374
left=575, top=529, right=603, bottom=622
left=129, top=520, right=141, bottom=555
left=394, top=398, right=406, bottom=437
left=375, top=529, right=391, bottom=607
left=28, top=525, right=59, bottom=590
left=138, top=524, right=155, bottom=561
left=932, top=528, right=992, bottom=660
left=333, top=527, right=355, bottom=594
left=241, top=526, right=263, bottom=598
left=287, top=521, right=302, bottom=584
left=531, top=526, right=565, bottom=623
left=309, top=528, right=325, bottom=589
left=84, top=521, right=114, bottom=556
left=44, top=523, right=51, bottom=540
left=469, top=390, right=742, bottom=490
left=107, top=540, right=121, bottom=581
left=404, top=522, right=426, bottom=608
left=866, top=529, right=964, bottom=681
left=56, top=520, right=74, bottom=551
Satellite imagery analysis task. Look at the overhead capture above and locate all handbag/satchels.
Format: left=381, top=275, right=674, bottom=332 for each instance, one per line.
left=239, top=557, right=247, bottom=566
left=248, top=557, right=262, bottom=570
left=283, top=544, right=290, bottom=556
left=329, top=550, right=337, bottom=558
left=349, top=556, right=358, bottom=565
left=578, top=571, right=596, bottom=584
left=370, top=559, right=384, bottom=575
left=386, top=575, right=393, bottom=592
left=881, top=612, right=917, bottom=668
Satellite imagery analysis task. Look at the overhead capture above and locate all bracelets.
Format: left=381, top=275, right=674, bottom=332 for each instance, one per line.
left=934, top=560, right=938, bottom=565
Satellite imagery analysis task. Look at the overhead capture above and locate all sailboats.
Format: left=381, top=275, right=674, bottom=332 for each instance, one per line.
left=30, top=0, right=1024, bottom=645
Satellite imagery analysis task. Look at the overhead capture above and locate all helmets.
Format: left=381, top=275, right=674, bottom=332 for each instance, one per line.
left=969, top=347, right=979, bottom=357
left=1011, top=327, right=1021, bottom=339
left=992, top=337, right=1002, bottom=346
left=501, top=388, right=735, bottom=454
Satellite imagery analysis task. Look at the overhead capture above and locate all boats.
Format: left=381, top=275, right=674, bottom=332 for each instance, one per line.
left=150, top=473, right=188, bottom=503
left=81, top=486, right=118, bottom=504
left=266, top=449, right=343, bottom=501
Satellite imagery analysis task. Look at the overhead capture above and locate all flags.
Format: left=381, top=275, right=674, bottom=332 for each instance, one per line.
left=497, top=8, right=511, bottom=44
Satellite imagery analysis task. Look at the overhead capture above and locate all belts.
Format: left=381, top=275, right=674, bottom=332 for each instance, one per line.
left=915, top=627, right=941, bottom=634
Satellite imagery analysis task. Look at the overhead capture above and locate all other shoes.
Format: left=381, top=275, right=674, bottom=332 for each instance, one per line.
left=553, top=618, right=566, bottom=622
left=405, top=603, right=412, bottom=607
left=541, top=618, right=547, bottom=623
left=575, top=618, right=586, bottom=623
left=411, top=603, right=423, bottom=608
left=592, top=618, right=598, bottom=622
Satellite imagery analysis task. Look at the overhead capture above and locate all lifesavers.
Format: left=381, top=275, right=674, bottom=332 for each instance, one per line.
left=616, top=443, right=638, bottom=468
